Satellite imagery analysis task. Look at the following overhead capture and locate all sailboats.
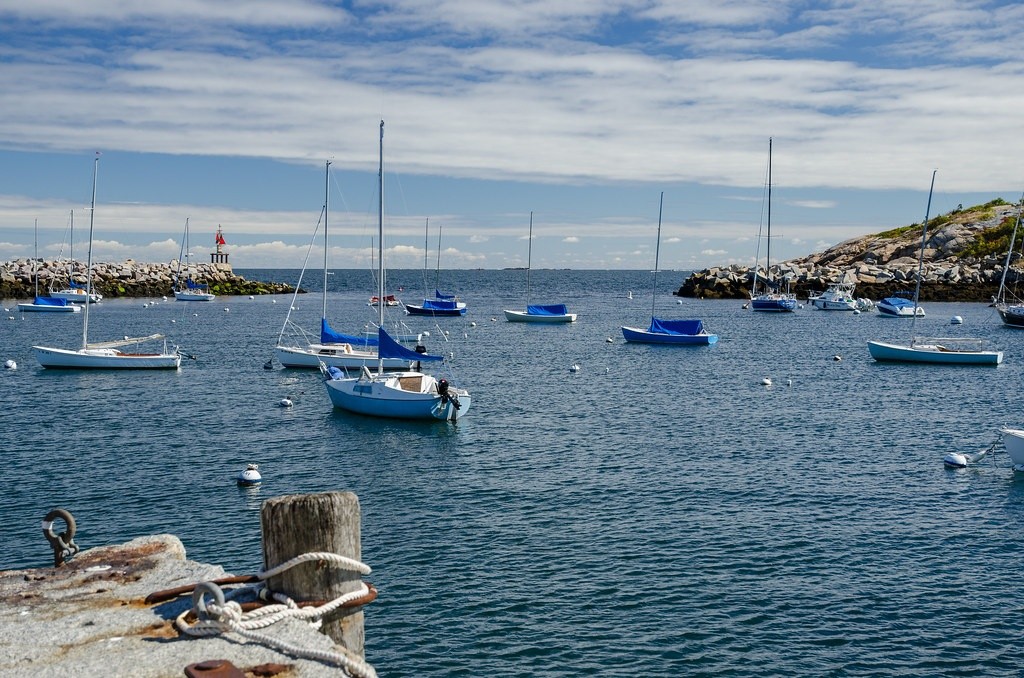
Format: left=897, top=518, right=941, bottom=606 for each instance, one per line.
left=620, top=192, right=718, bottom=345
left=867, top=170, right=1005, bottom=365
left=992, top=191, right=1024, bottom=328
left=504, top=211, right=577, bottom=322
left=748, top=137, right=797, bottom=312
left=31, top=158, right=196, bottom=371
left=324, top=118, right=471, bottom=423
left=174, top=217, right=216, bottom=301
left=405, top=218, right=468, bottom=317
left=47, top=209, right=103, bottom=303
left=18, top=218, right=82, bottom=312
left=274, top=159, right=416, bottom=371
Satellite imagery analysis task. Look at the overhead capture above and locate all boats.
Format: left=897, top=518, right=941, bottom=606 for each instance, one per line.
left=806, top=269, right=874, bottom=311
left=876, top=291, right=926, bottom=318
left=995, top=426, right=1024, bottom=470
